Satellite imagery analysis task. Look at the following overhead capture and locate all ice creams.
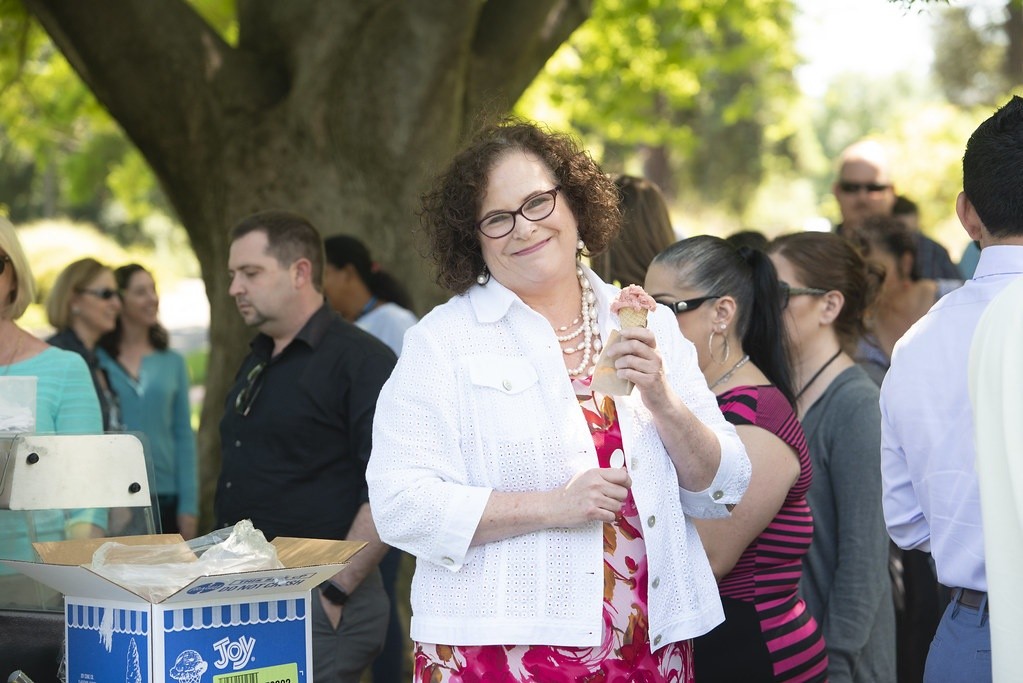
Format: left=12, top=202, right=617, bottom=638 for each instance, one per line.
left=611, top=283, right=657, bottom=342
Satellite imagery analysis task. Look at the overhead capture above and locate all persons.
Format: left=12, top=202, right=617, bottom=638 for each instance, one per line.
left=880, top=96, right=1023, bottom=683
left=0, top=217, right=200, bottom=606
left=214, top=207, right=419, bottom=683
left=591, top=158, right=963, bottom=683
left=365, top=113, right=752, bottom=683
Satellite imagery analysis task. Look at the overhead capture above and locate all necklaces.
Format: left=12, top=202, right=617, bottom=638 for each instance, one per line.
left=794, top=348, right=842, bottom=401
left=552, top=263, right=601, bottom=378
left=2, top=330, right=25, bottom=376
left=709, top=355, right=750, bottom=389
left=349, top=295, right=378, bottom=322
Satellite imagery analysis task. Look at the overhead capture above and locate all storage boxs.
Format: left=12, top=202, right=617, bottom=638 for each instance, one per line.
left=0, top=534, right=370, bottom=682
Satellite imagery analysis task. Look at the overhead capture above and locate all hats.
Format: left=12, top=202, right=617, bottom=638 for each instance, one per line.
left=0, top=215, right=34, bottom=319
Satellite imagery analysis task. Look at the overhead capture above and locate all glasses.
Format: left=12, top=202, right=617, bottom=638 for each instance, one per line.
left=234, top=359, right=266, bottom=417
left=76, top=286, right=120, bottom=300
left=0, top=256, right=10, bottom=273
left=658, top=295, right=722, bottom=318
left=477, top=185, right=562, bottom=239
left=840, top=181, right=891, bottom=193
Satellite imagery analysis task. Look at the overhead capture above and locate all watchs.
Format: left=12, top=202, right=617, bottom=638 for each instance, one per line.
left=316, top=578, right=348, bottom=606
